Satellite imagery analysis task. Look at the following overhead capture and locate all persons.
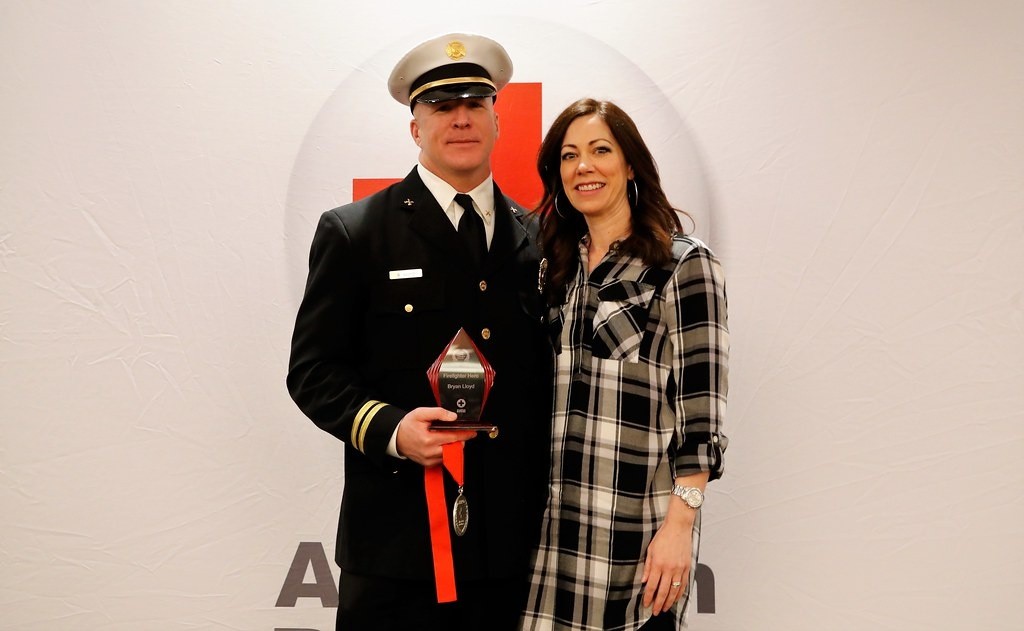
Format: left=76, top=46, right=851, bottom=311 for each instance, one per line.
left=284, top=31, right=545, bottom=631
left=516, top=97, right=729, bottom=631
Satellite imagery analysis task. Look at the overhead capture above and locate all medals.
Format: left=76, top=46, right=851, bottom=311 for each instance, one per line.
left=453, top=494, right=469, bottom=536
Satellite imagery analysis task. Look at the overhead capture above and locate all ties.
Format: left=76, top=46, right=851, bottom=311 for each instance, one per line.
left=454, top=194, right=489, bottom=260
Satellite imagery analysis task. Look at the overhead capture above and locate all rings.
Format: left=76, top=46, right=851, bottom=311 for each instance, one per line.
left=671, top=581, right=681, bottom=586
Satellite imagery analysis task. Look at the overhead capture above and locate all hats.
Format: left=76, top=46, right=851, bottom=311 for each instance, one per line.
left=387, top=33, right=514, bottom=115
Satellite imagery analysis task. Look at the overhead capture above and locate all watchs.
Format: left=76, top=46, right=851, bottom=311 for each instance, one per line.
left=670, top=484, right=706, bottom=510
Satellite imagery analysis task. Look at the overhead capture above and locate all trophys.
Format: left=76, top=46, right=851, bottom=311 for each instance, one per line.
left=426, top=326, right=500, bottom=433
